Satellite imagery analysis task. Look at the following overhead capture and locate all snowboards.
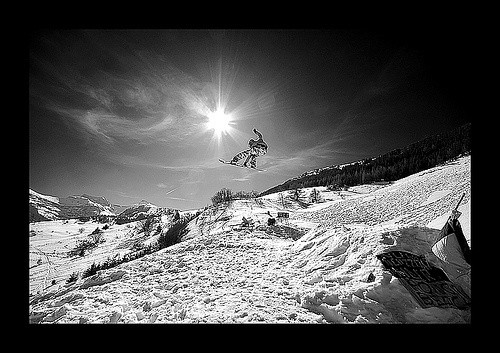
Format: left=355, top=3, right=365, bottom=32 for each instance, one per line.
left=214, top=157, right=267, bottom=172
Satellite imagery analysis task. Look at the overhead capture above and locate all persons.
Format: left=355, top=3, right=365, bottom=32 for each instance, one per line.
left=229, top=127, right=269, bottom=170
